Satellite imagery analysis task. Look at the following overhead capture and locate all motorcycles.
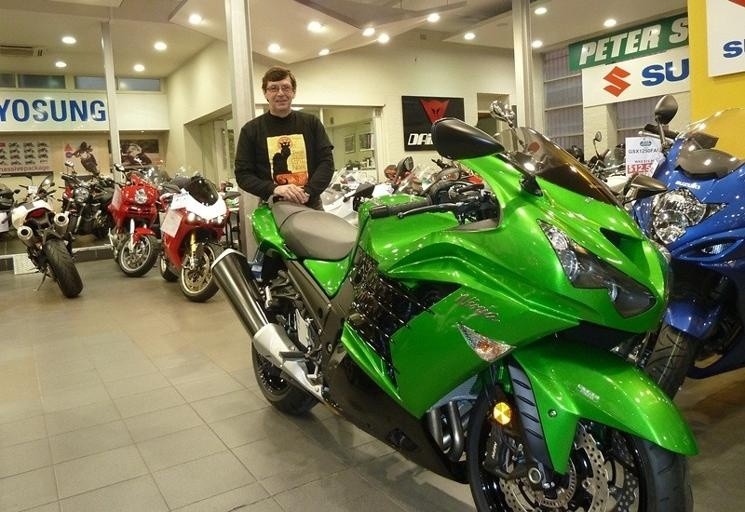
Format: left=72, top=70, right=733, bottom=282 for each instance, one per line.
left=323, top=98, right=654, bottom=221
left=0, top=174, right=84, bottom=298
left=620, top=95, right=745, bottom=400
left=209, top=98, right=696, bottom=512
left=52, top=151, right=242, bottom=302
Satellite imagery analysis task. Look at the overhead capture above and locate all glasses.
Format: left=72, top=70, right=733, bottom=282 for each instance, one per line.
left=265, top=84, right=291, bottom=92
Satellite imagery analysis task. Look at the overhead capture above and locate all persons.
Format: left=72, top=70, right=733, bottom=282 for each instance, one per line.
left=235, top=66, right=335, bottom=209
